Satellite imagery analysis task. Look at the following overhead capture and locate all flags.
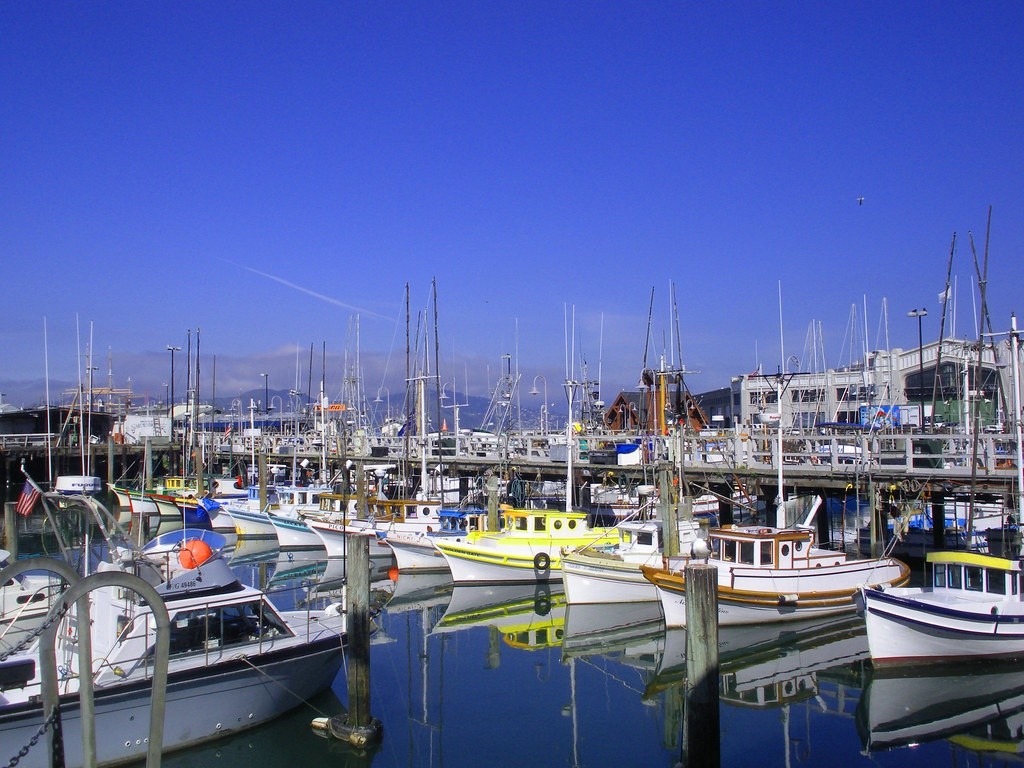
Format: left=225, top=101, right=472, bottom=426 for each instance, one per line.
left=14, top=477, right=44, bottom=518
left=225, top=426, right=231, bottom=439
left=938, top=286, right=952, bottom=303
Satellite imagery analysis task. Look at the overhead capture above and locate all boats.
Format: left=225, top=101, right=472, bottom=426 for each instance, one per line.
left=562, top=596, right=670, bottom=768
left=0, top=309, right=354, bottom=768
left=857, top=662, right=1024, bottom=768
left=643, top=612, right=870, bottom=768
left=642, top=280, right=910, bottom=626
left=435, top=307, right=630, bottom=584
left=425, top=579, right=569, bottom=676
left=860, top=313, right=1024, bottom=659
left=219, top=538, right=452, bottom=631
left=557, top=293, right=708, bottom=603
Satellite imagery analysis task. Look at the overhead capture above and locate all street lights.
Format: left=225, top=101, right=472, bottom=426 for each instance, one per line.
left=908, top=308, right=928, bottom=433
left=167, top=346, right=181, bottom=432
left=784, top=355, right=803, bottom=436
left=439, top=381, right=456, bottom=436
left=260, top=372, right=268, bottom=412
left=267, top=392, right=283, bottom=437
left=373, top=385, right=389, bottom=435
left=530, top=374, right=548, bottom=436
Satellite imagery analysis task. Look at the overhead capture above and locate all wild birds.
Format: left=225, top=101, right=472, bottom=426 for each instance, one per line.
left=923, top=308, right=927, bottom=315
left=912, top=309, right=917, bottom=312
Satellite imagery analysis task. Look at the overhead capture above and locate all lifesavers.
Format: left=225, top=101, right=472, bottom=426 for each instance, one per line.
left=534, top=552, right=551, bottom=570
left=534, top=599, right=551, bottom=617
left=810, top=456, right=819, bottom=465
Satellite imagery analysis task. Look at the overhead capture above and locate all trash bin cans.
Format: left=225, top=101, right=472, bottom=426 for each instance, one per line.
left=550, top=444, right=575, bottom=462
left=913, top=439, right=943, bottom=468
left=616, top=444, right=640, bottom=465
left=372, top=446, right=388, bottom=457
left=444, top=437, right=456, bottom=456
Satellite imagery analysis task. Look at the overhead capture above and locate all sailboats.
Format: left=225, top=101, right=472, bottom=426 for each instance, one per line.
left=108, top=290, right=499, bottom=571
left=542, top=273, right=1013, bottom=531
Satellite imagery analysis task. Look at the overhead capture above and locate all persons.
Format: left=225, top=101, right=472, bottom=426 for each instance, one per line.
left=306, top=469, right=312, bottom=477
